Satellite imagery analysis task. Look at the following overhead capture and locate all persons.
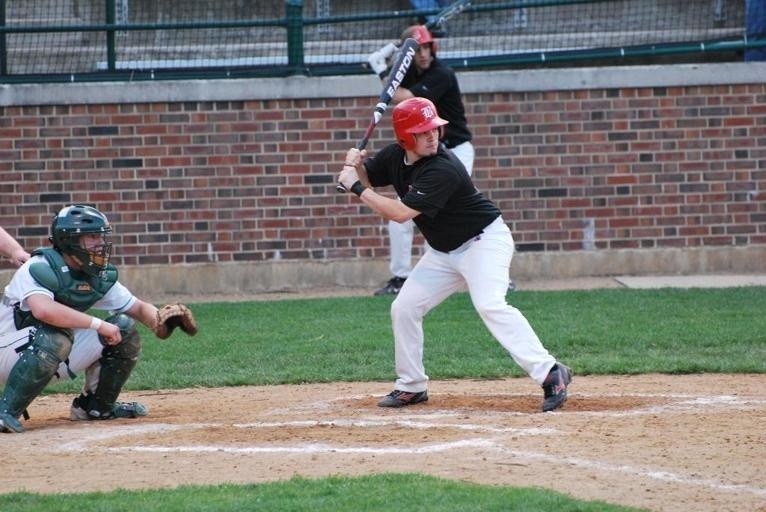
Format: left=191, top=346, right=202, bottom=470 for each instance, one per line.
left=368, top=24, right=476, bottom=295
left=0, top=203, right=197, bottom=435
left=337, top=97, right=572, bottom=412
left=0, top=225, right=31, bottom=268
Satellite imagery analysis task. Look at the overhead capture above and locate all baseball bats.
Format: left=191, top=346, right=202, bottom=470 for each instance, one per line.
left=361, top=1, right=474, bottom=70
left=334, top=39, right=422, bottom=193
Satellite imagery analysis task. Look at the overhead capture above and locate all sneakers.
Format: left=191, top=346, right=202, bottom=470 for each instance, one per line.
left=373, top=278, right=407, bottom=295
left=378, top=390, right=430, bottom=406
left=71, top=398, right=145, bottom=419
left=542, top=364, right=573, bottom=410
left=0, top=412, right=24, bottom=434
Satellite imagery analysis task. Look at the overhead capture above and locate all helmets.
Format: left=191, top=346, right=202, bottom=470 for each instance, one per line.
left=403, top=26, right=437, bottom=57
left=394, top=97, right=448, bottom=148
left=51, top=204, right=112, bottom=233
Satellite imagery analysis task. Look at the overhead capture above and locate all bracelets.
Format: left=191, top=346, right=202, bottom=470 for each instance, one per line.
left=89, top=316, right=102, bottom=330
left=348, top=180, right=367, bottom=197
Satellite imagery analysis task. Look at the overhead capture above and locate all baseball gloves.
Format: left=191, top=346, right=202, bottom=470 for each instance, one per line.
left=154, top=302, right=198, bottom=339
